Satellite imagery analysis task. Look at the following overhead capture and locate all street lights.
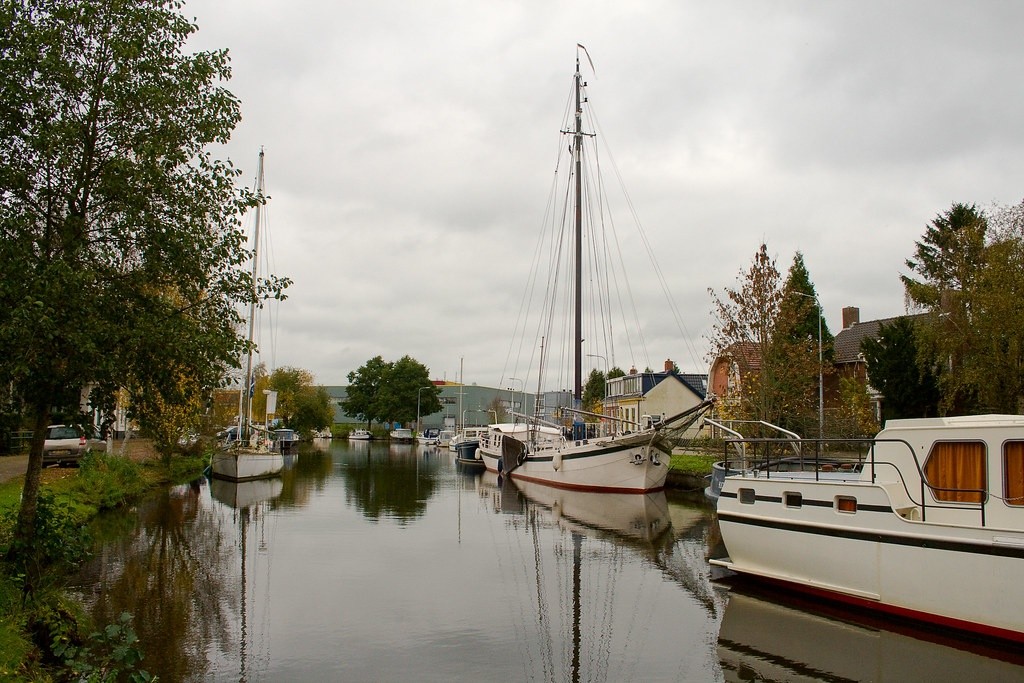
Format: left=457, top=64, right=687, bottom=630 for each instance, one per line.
left=790, top=289, right=826, bottom=451
left=509, top=378, right=524, bottom=423
left=586, top=353, right=607, bottom=416
left=417, top=386, right=431, bottom=433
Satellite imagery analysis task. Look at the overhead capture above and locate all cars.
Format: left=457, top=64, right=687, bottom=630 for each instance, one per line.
left=42, top=424, right=108, bottom=468
left=215, top=426, right=255, bottom=440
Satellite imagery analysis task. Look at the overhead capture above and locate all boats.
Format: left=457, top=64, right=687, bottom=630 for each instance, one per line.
left=436, top=430, right=455, bottom=447
left=416, top=437, right=437, bottom=445
left=454, top=409, right=498, bottom=466
left=209, top=142, right=285, bottom=482
left=390, top=427, right=414, bottom=441
left=347, top=428, right=374, bottom=440
left=478, top=40, right=674, bottom=494
left=271, top=428, right=295, bottom=449
left=702, top=412, right=1024, bottom=643
